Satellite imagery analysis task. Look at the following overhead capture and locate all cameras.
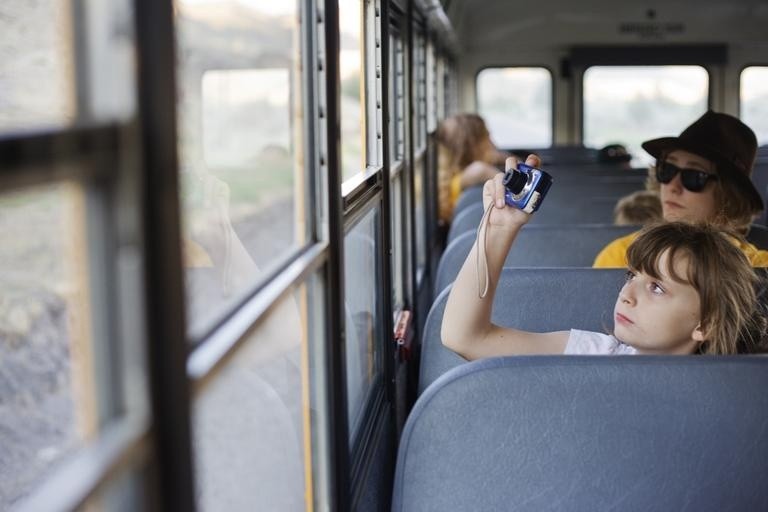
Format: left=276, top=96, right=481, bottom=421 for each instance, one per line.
left=494, top=164, right=552, bottom=214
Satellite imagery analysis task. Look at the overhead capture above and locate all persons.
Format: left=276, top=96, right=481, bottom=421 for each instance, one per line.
left=598, top=144, right=633, bottom=171
left=439, top=112, right=500, bottom=224
left=590, top=108, right=768, bottom=271
left=439, top=151, right=768, bottom=361
left=186, top=161, right=264, bottom=337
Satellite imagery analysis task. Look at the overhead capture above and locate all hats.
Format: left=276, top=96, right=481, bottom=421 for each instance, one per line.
left=597, top=145, right=631, bottom=163
left=642, top=109, right=764, bottom=212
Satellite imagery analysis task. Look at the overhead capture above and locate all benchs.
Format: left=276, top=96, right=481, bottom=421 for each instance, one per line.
left=391, top=353, right=767, bottom=510
left=416, top=148, right=768, bottom=388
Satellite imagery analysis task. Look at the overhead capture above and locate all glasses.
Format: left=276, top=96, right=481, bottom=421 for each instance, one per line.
left=655, top=159, right=722, bottom=194
